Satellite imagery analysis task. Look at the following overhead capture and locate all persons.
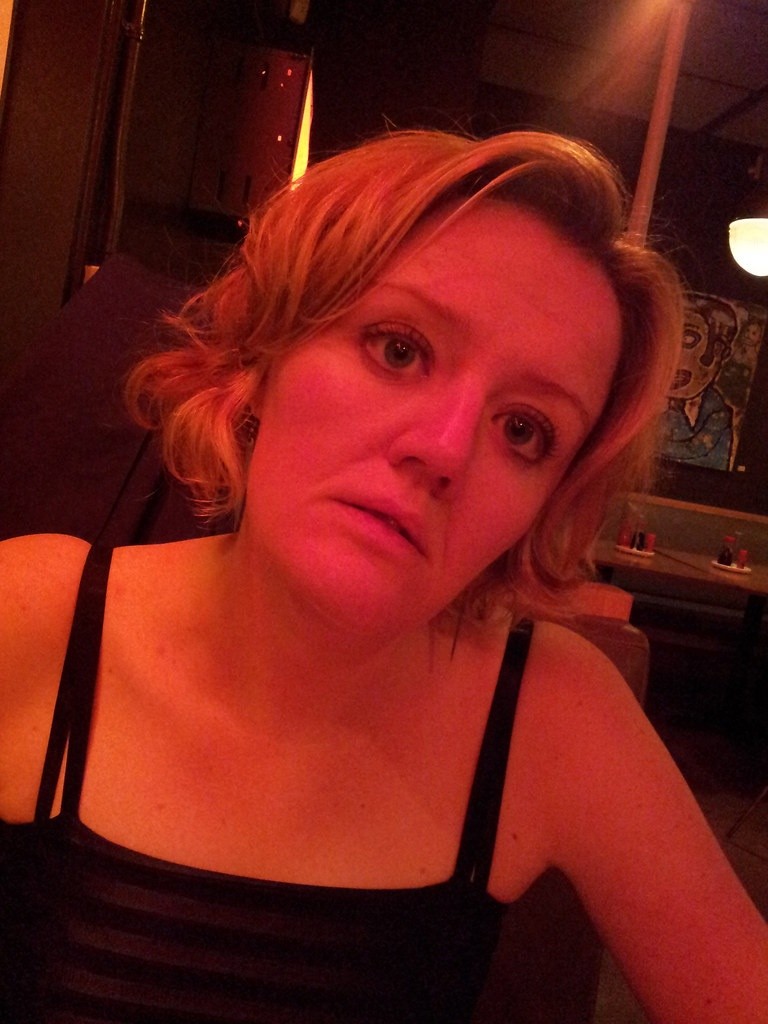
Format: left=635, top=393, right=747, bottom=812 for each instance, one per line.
left=0, top=131, right=768, bottom=1024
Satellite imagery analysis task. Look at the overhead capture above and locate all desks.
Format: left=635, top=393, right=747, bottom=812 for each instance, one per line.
left=589, top=540, right=768, bottom=748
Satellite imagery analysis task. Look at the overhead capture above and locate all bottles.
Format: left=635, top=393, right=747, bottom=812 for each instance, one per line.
left=717, top=531, right=748, bottom=569
left=618, top=502, right=655, bottom=552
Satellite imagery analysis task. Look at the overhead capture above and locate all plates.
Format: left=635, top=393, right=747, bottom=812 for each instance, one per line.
left=614, top=543, right=654, bottom=557
left=711, top=560, right=751, bottom=574
